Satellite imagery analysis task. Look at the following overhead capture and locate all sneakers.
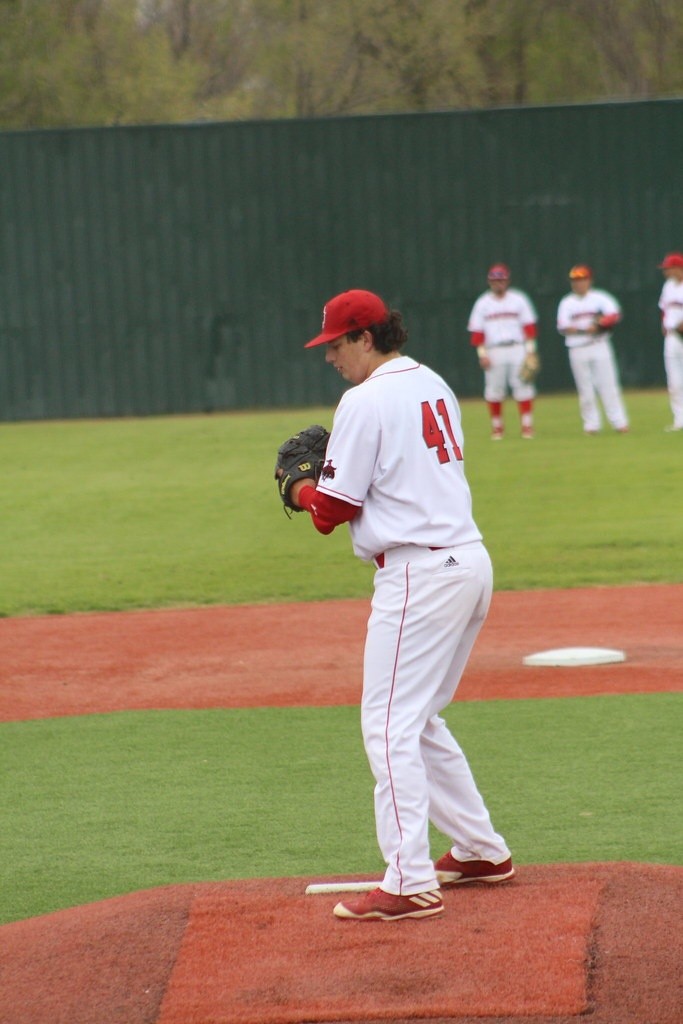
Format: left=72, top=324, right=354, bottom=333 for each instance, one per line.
left=330, top=886, right=445, bottom=922
left=434, top=845, right=515, bottom=888
left=491, top=423, right=534, bottom=440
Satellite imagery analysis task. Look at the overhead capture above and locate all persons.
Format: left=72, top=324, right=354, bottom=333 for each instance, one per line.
left=275, top=289, right=518, bottom=922
left=554, top=268, right=631, bottom=433
left=656, top=255, right=683, bottom=434
left=468, top=268, right=542, bottom=442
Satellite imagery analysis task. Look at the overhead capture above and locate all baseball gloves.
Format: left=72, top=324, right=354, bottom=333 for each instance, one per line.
left=271, top=423, right=332, bottom=523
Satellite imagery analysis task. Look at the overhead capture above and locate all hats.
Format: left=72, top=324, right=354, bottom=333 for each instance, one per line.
left=302, top=287, right=390, bottom=349
left=487, top=266, right=511, bottom=283
left=568, top=266, right=591, bottom=279
left=656, top=254, right=682, bottom=270
